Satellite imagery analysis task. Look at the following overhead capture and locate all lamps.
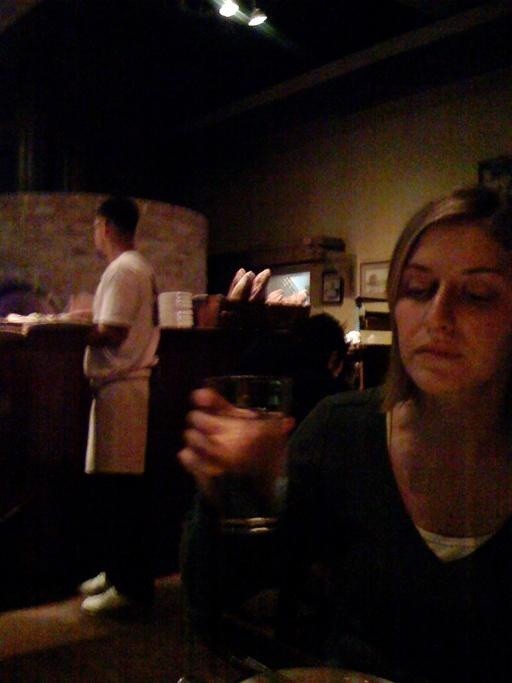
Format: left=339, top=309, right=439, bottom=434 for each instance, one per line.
left=248, top=7, right=267, bottom=27
left=213, top=0, right=239, bottom=18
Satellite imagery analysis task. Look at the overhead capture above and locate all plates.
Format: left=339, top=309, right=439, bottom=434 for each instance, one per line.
left=154, top=291, right=192, bottom=326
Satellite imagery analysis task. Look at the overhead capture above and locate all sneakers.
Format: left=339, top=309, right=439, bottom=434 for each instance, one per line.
left=79, top=570, right=111, bottom=594
left=80, top=585, right=133, bottom=616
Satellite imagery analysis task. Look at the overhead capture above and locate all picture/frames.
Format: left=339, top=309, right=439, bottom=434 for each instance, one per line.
left=320, top=271, right=344, bottom=304
left=359, top=260, right=391, bottom=299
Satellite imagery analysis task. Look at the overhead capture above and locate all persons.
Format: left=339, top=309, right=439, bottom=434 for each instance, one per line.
left=78, top=195, right=160, bottom=618
left=177, top=184, right=512, bottom=683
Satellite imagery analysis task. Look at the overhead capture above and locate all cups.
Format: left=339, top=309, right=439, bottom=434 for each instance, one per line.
left=208, top=375, right=292, bottom=533
